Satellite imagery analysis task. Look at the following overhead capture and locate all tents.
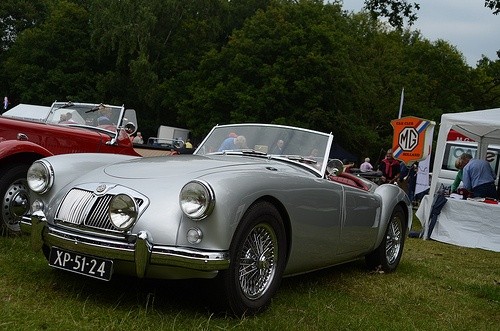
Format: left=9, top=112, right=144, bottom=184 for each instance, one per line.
left=3, top=104, right=87, bottom=129
left=423, top=108, right=500, bottom=252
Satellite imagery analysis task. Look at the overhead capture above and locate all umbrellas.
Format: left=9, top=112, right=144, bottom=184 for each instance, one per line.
left=428, top=184, right=450, bottom=239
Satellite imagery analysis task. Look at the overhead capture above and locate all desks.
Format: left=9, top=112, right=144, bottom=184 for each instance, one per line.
left=416, top=194, right=500, bottom=254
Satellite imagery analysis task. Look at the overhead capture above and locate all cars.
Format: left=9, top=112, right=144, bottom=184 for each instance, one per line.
left=0, top=99, right=195, bottom=239
left=42, top=124, right=417, bottom=320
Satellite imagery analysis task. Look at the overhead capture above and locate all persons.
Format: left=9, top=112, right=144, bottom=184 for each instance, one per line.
left=59, top=113, right=288, bottom=154
left=344, top=149, right=499, bottom=205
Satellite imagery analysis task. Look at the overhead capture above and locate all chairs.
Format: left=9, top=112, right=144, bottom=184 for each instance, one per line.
left=329, top=173, right=367, bottom=191
